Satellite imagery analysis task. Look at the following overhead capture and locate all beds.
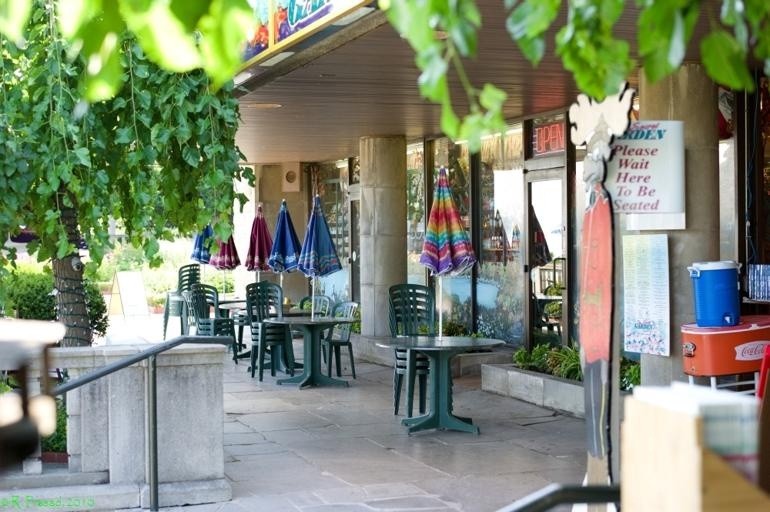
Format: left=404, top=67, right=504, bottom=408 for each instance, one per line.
left=244, top=202, right=274, bottom=283
left=529, top=200, right=553, bottom=270
left=297, top=194, right=343, bottom=323
left=209, top=232, right=241, bottom=301
left=266, top=198, right=302, bottom=289
left=419, top=164, right=478, bottom=342
left=191, top=222, right=214, bottom=284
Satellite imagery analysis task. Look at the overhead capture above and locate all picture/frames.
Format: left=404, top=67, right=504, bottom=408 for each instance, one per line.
left=374, top=337, right=506, bottom=437
left=263, top=317, right=361, bottom=390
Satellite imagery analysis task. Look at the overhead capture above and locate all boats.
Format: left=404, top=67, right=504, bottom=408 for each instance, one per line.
left=281, top=163, right=302, bottom=193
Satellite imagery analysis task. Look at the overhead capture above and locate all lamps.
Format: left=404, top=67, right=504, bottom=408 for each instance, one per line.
left=388, top=284, right=453, bottom=418
left=162, top=263, right=360, bottom=381
